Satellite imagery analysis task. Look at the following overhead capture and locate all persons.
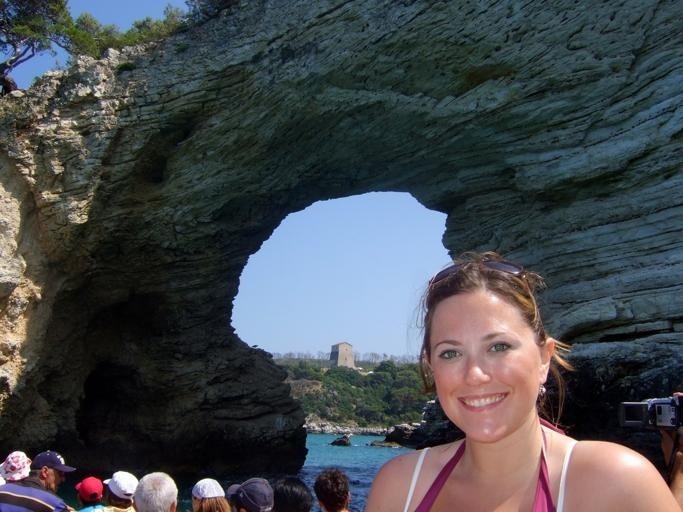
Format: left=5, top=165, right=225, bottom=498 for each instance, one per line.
left=274, top=477, right=314, bottom=511
left=313, top=469, right=353, bottom=512
left=190, top=477, right=231, bottom=512
left=75, top=475, right=105, bottom=511
left=132, top=471, right=178, bottom=512
left=658, top=391, right=683, bottom=512
left=101, top=471, right=138, bottom=511
left=1, top=448, right=78, bottom=511
left=361, top=248, right=683, bottom=512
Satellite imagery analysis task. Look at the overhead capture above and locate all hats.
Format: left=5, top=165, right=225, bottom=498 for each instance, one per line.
left=102, top=470, right=140, bottom=500
left=73, top=476, right=103, bottom=502
left=192, top=478, right=225, bottom=497
left=30, top=449, right=77, bottom=472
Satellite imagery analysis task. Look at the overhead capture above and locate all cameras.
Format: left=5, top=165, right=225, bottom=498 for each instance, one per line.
left=619, top=396, right=683, bottom=431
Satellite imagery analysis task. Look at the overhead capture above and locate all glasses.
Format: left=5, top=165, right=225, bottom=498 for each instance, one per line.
left=427, top=260, right=546, bottom=339
